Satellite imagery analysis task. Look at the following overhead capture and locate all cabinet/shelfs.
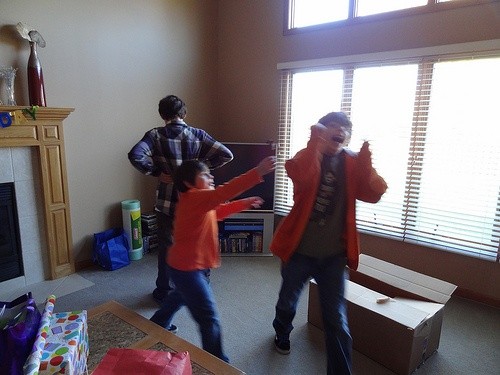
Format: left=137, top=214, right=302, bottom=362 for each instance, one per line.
left=217, top=212, right=274, bottom=257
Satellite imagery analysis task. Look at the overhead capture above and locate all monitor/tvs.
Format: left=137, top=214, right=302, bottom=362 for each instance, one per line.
left=210, top=142, right=277, bottom=213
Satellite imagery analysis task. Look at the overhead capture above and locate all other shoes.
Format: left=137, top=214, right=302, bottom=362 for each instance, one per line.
left=274, top=333, right=291, bottom=354
left=152, top=288, right=168, bottom=308
left=168, top=324, right=178, bottom=333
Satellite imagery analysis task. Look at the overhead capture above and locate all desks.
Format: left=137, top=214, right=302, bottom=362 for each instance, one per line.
left=86, top=299, right=247, bottom=375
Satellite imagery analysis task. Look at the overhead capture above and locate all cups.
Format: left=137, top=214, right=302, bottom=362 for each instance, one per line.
left=0, top=67, right=17, bottom=106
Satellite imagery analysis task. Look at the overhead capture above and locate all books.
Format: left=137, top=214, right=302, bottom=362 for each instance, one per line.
left=141, top=211, right=159, bottom=251
left=218, top=233, right=263, bottom=253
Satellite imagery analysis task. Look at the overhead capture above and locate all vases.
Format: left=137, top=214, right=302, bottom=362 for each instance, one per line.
left=27, top=41, right=47, bottom=107
left=4, top=77, right=17, bottom=106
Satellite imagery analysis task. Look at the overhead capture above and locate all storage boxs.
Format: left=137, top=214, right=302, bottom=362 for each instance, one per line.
left=23, top=309, right=90, bottom=375
left=307, top=254, right=459, bottom=375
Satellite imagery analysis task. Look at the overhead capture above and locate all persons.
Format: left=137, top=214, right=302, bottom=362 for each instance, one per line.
left=149, top=156, right=276, bottom=363
left=269, top=112, right=389, bottom=375
left=128, top=95, right=233, bottom=299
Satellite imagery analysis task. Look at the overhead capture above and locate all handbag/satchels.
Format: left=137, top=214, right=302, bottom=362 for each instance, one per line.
left=0, top=292, right=43, bottom=375
left=90, top=347, right=192, bottom=375
left=92, top=227, right=131, bottom=272
